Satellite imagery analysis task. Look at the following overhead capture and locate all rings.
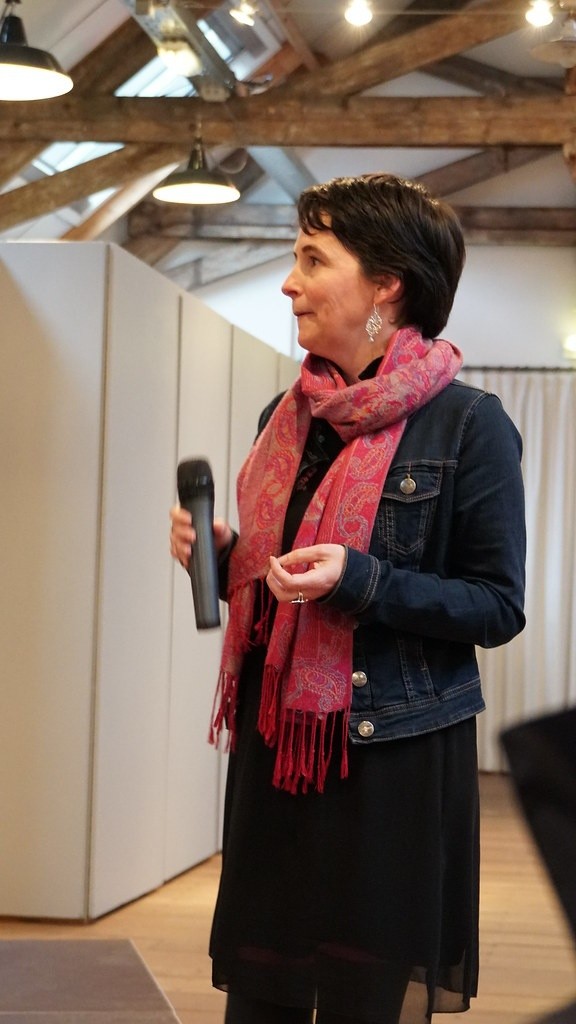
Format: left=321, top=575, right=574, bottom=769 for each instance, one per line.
left=288, top=592, right=308, bottom=605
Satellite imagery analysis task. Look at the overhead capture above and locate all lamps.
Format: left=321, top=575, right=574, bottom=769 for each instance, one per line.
left=152, top=139, right=240, bottom=206
left=0, top=13, right=74, bottom=102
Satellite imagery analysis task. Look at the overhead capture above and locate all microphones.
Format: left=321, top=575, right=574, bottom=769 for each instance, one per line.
left=177, top=459, right=222, bottom=629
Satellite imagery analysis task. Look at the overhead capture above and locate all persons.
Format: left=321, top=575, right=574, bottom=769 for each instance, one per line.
left=169, top=174, right=525, bottom=1024
